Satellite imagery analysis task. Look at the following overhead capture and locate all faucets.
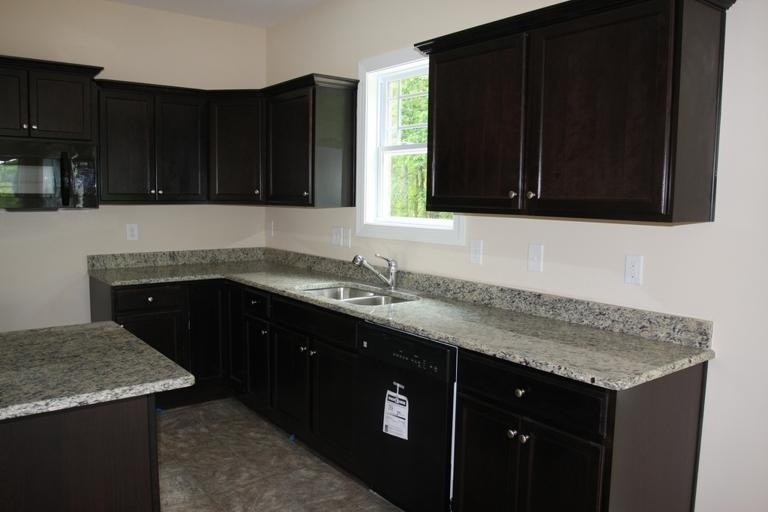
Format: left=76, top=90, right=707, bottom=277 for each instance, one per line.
left=352, top=254, right=398, bottom=290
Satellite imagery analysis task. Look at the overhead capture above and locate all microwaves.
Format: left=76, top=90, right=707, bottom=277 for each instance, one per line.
left=1, top=136, right=98, bottom=211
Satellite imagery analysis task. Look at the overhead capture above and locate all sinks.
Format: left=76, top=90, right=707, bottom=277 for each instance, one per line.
left=336, top=294, right=421, bottom=306
left=300, top=283, right=378, bottom=299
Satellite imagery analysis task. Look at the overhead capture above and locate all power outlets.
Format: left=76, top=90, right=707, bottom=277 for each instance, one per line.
left=624, top=255, right=644, bottom=285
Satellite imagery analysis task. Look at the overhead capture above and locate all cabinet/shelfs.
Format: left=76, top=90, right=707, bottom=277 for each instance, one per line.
left=89, top=275, right=229, bottom=410
left=414, top=0, right=735, bottom=223
left=263, top=73, right=359, bottom=208
left=0, top=54, right=105, bottom=208
left=224, top=277, right=273, bottom=418
left=210, top=89, right=265, bottom=204
left=452, top=349, right=613, bottom=509
left=272, top=295, right=360, bottom=473
left=95, top=79, right=209, bottom=204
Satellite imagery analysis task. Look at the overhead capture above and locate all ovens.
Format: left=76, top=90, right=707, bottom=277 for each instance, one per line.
left=354, top=320, right=456, bottom=511
